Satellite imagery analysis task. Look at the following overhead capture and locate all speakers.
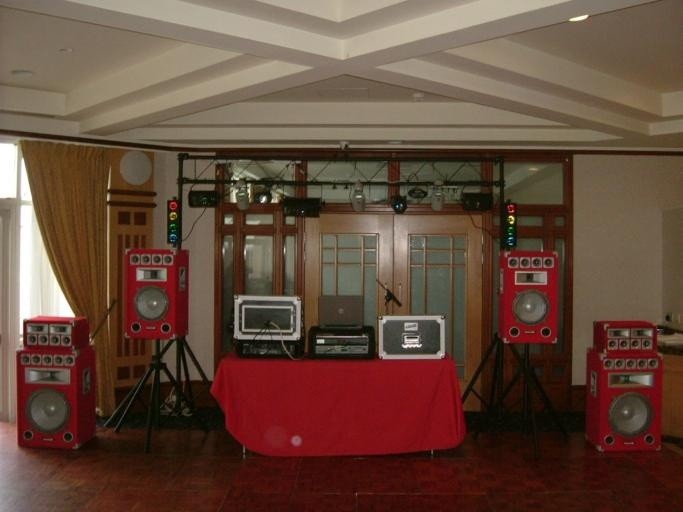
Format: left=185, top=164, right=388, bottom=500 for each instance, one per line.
left=23, top=316, right=89, bottom=350
left=586, top=347, right=664, bottom=452
left=124, top=248, right=188, bottom=340
left=593, top=320, right=657, bottom=354
left=497, top=251, right=560, bottom=345
left=16, top=345, right=96, bottom=450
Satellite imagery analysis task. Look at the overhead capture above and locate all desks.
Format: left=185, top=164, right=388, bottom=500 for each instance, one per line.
left=650, top=325, right=681, bottom=440
left=210, top=350, right=466, bottom=460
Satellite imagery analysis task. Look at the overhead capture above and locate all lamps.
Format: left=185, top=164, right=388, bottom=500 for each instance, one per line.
left=231, top=178, right=447, bottom=217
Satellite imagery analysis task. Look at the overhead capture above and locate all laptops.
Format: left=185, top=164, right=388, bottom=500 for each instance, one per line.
left=318, top=295, right=364, bottom=330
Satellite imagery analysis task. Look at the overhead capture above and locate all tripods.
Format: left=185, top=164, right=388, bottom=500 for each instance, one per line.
left=103, top=337, right=211, bottom=464
left=461, top=335, right=563, bottom=460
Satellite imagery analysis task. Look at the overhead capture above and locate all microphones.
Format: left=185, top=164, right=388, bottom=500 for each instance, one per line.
left=385, top=288, right=402, bottom=308
